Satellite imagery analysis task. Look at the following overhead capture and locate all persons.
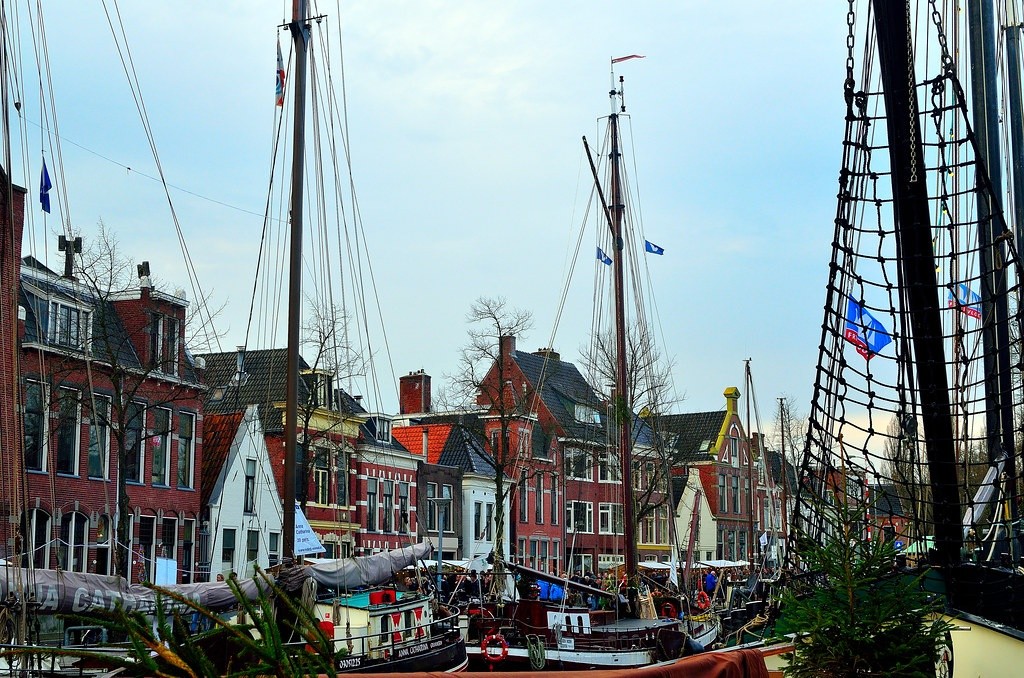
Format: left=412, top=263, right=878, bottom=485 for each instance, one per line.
left=210, top=572, right=237, bottom=630
left=537, top=570, right=628, bottom=610
left=636, top=566, right=773, bottom=599
left=62, top=618, right=82, bottom=645
left=398, top=569, right=498, bottom=604
left=191, top=576, right=208, bottom=631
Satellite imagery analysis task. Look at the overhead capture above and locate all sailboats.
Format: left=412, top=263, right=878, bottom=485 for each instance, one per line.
left=0, top=0, right=1024, bottom=678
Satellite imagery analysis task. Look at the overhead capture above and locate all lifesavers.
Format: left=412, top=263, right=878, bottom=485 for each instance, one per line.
left=620, top=576, right=627, bottom=589
left=661, top=603, right=674, bottom=616
left=697, top=591, right=708, bottom=609
left=481, top=634, right=508, bottom=662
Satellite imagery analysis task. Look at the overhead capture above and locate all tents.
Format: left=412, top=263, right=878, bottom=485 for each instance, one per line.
left=640, top=560, right=760, bottom=569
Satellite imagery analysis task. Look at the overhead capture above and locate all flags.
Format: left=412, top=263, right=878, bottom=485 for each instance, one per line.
left=948, top=281, right=981, bottom=319
left=597, top=248, right=612, bottom=265
left=853, top=294, right=892, bottom=361
left=646, top=241, right=664, bottom=255
left=759, top=532, right=768, bottom=545
